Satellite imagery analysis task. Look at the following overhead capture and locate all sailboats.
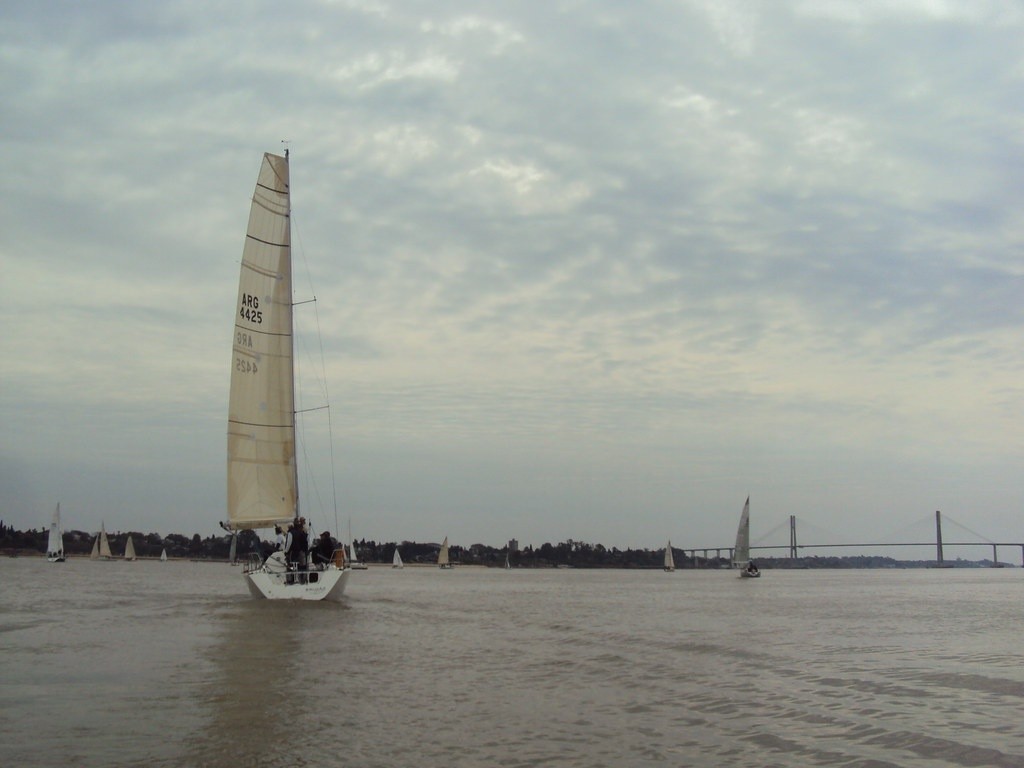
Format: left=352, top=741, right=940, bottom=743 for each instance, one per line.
left=87, top=520, right=113, bottom=561
left=45, top=500, right=66, bottom=562
left=438, top=536, right=452, bottom=569
left=731, top=494, right=761, bottom=578
left=124, top=536, right=138, bottom=562
left=504, top=552, right=511, bottom=569
left=347, top=531, right=369, bottom=570
left=161, top=548, right=168, bottom=562
left=229, top=529, right=240, bottom=566
left=662, top=540, right=675, bottom=573
left=393, top=547, right=404, bottom=570
left=219, top=146, right=351, bottom=601
